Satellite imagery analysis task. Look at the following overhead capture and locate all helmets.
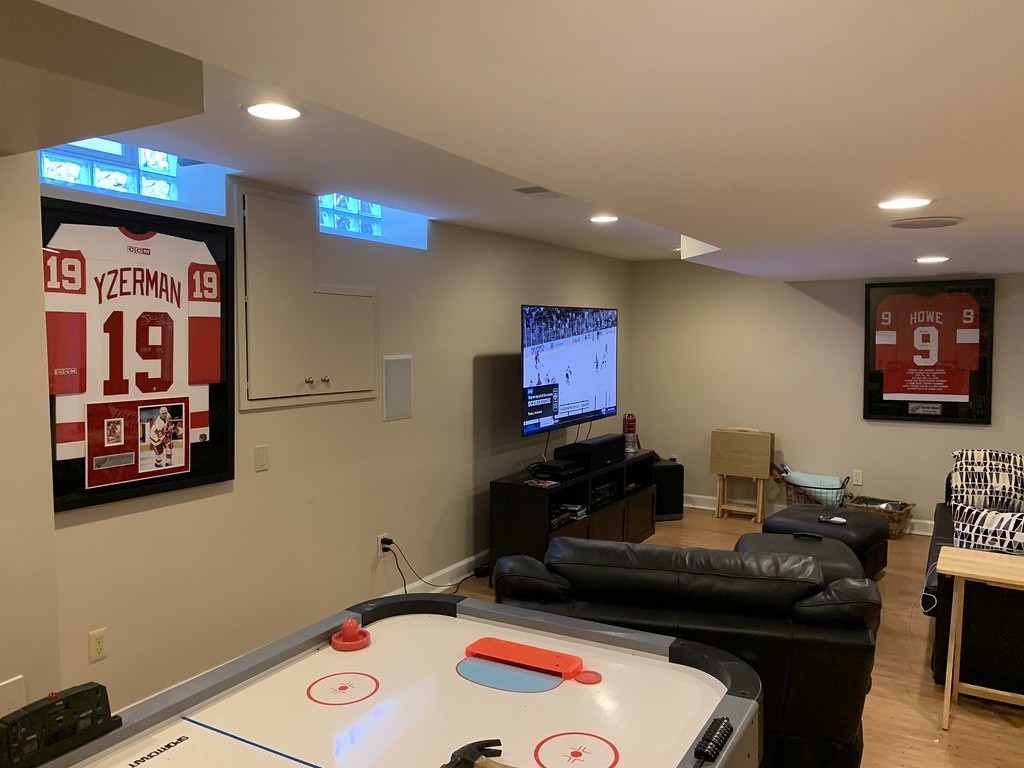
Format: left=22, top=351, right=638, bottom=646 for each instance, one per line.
left=160, top=407, right=168, bottom=414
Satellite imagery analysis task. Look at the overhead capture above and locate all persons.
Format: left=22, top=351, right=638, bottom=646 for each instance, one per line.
left=109, top=407, right=175, bottom=468
left=523, top=306, right=617, bottom=387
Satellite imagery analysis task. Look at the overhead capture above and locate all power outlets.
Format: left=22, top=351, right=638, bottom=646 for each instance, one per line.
left=378, top=533, right=389, bottom=558
left=853, top=469, right=864, bottom=486
left=88, top=627, right=108, bottom=665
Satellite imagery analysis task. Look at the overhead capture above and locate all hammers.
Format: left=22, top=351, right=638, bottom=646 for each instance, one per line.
left=439, top=737, right=517, bottom=768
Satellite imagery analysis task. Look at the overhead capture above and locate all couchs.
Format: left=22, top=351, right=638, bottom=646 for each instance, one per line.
left=494, top=535, right=884, bottom=768
left=922, top=470, right=1024, bottom=694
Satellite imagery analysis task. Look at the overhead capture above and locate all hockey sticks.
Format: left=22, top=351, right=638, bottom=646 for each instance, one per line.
left=770, top=463, right=829, bottom=505
left=169, top=432, right=172, bottom=466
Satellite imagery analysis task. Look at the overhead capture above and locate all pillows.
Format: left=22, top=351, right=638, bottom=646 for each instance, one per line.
left=950, top=448, right=1024, bottom=513
left=950, top=499, right=1024, bottom=556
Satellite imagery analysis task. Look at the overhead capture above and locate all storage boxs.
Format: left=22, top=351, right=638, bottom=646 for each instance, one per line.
left=775, top=476, right=850, bottom=508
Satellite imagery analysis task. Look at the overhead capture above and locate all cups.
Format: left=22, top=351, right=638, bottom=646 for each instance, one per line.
left=625, top=434, right=641, bottom=452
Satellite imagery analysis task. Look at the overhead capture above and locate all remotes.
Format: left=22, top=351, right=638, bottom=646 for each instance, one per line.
left=794, top=532, right=823, bottom=541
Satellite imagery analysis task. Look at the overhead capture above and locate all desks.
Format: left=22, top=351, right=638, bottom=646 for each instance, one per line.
left=934, top=545, right=1024, bottom=730
left=36, top=593, right=768, bottom=768
left=708, top=427, right=775, bottom=523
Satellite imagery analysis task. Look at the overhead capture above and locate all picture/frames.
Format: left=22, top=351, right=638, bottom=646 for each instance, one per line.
left=862, top=278, right=992, bottom=426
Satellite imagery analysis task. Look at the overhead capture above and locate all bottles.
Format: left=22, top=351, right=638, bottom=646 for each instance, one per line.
left=623, top=414, right=636, bottom=434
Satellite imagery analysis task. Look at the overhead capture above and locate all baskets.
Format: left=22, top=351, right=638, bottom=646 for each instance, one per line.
left=784, top=471, right=851, bottom=507
left=844, top=492, right=916, bottom=535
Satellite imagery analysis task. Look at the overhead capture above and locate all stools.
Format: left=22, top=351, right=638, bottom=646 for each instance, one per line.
left=737, top=531, right=864, bottom=578
left=763, top=503, right=889, bottom=579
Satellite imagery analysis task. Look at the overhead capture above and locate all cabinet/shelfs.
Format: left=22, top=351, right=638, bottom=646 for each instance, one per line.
left=654, top=457, right=685, bottom=521
left=488, top=448, right=658, bottom=587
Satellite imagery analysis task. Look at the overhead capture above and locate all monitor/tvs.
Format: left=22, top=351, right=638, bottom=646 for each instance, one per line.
left=520, top=305, right=617, bottom=435
